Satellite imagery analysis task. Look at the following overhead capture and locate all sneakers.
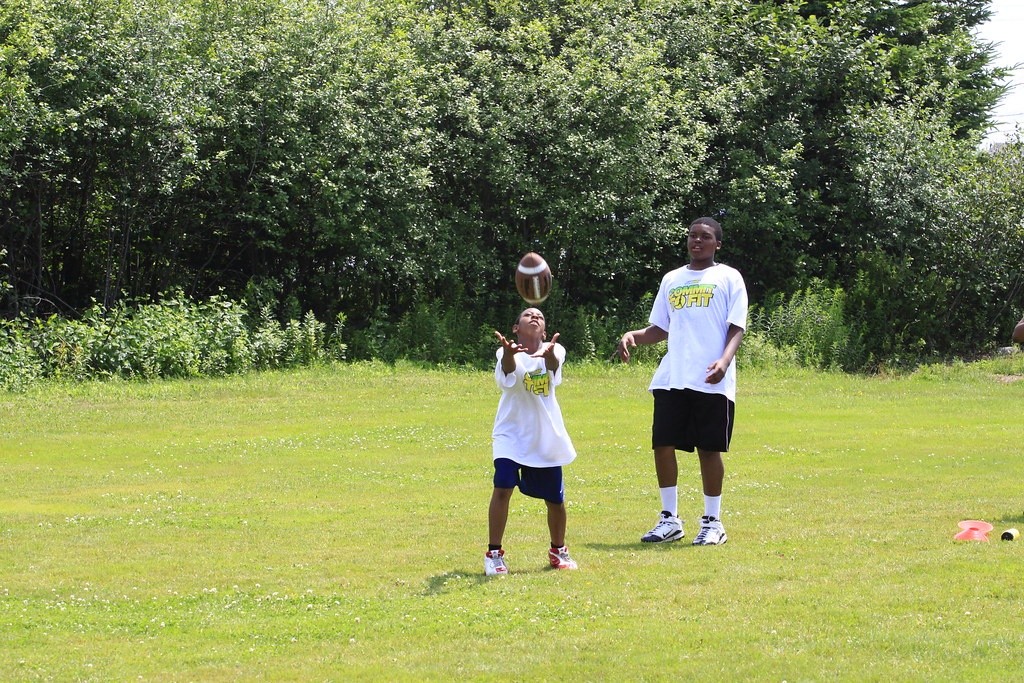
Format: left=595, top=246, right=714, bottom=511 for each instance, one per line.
left=641, top=511, right=685, bottom=543
left=484, top=550, right=509, bottom=575
left=548, top=546, right=577, bottom=569
left=692, top=516, right=727, bottom=545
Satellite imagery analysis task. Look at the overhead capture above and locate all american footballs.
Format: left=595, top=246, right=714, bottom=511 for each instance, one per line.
left=515, top=252, right=552, bottom=305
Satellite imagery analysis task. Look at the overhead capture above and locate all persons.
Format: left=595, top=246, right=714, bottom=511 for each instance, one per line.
left=485, top=305, right=580, bottom=574
left=619, top=216, right=750, bottom=545
